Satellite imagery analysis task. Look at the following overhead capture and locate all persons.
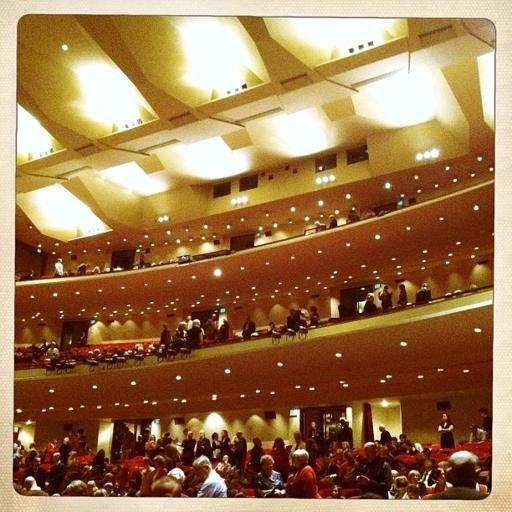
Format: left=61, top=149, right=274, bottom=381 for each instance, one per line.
left=87, top=349, right=103, bottom=360
left=242, top=315, right=255, bottom=340
left=348, top=207, right=360, bottom=221
left=329, top=214, right=337, bottom=228
left=54, top=258, right=64, bottom=277
left=77, top=263, right=90, bottom=275
left=267, top=305, right=320, bottom=336
left=338, top=282, right=432, bottom=319
left=14, top=408, right=492, bottom=496
left=140, top=247, right=150, bottom=267
left=160, top=313, right=230, bottom=349
left=15, top=338, right=61, bottom=363
